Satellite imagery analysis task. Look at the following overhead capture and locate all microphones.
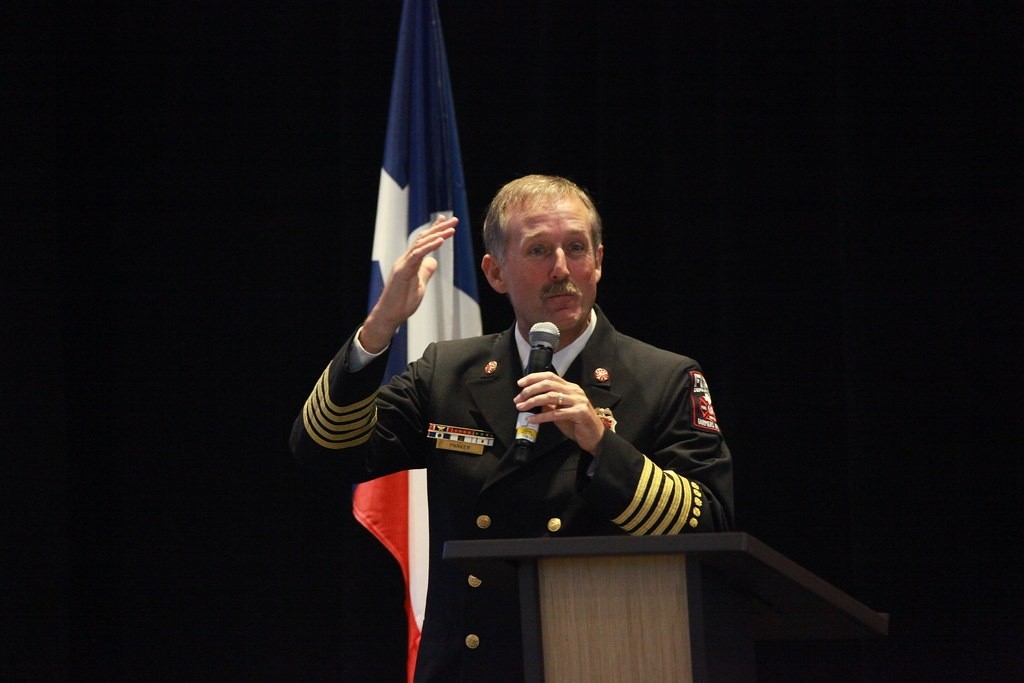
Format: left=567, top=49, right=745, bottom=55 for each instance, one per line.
left=513, top=322, right=561, bottom=465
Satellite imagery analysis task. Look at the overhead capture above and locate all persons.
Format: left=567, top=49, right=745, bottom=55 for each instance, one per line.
left=288, top=175, right=735, bottom=682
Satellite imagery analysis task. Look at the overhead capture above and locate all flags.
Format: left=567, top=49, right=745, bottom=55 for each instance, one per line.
left=354, top=0, right=484, bottom=683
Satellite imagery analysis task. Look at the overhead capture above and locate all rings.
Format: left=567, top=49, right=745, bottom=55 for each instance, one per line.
left=557, top=391, right=564, bottom=406
